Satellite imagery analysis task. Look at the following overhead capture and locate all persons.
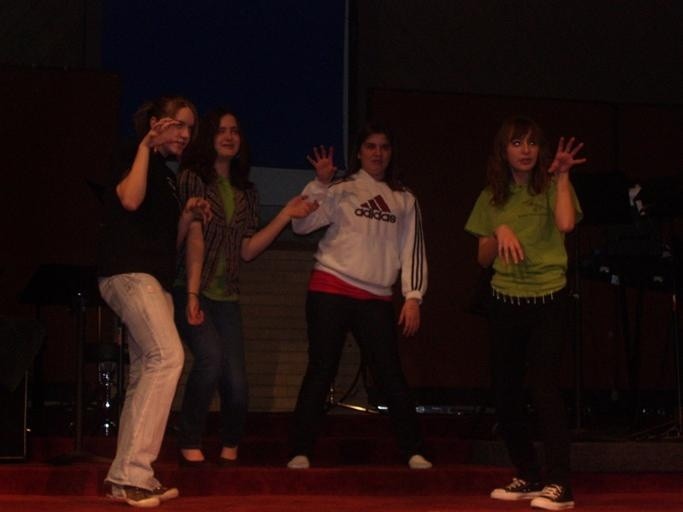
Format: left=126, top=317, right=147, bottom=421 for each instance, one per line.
left=95, top=93, right=214, bottom=508
left=170, top=105, right=323, bottom=465
left=285, top=118, right=437, bottom=469
left=461, top=111, right=588, bottom=510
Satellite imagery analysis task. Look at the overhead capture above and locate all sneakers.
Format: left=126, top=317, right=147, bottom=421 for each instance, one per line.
left=408, top=454, right=434, bottom=470
left=491, top=476, right=544, bottom=501
left=286, top=456, right=311, bottom=470
left=153, top=484, right=179, bottom=501
left=529, top=481, right=577, bottom=512
left=104, top=482, right=161, bottom=509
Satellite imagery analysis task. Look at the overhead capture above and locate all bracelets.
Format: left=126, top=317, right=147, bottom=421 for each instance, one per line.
left=186, top=290, right=202, bottom=302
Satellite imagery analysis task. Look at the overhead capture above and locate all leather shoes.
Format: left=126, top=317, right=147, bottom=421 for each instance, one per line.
left=179, top=448, right=205, bottom=461
left=221, top=442, right=239, bottom=460
